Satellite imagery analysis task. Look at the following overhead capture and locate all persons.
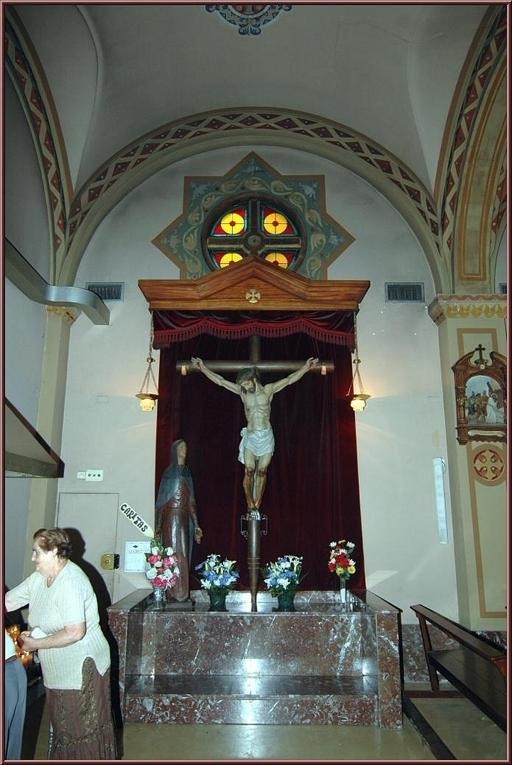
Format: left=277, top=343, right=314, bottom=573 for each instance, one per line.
left=5, top=529, right=121, bottom=763
left=193, top=356, right=319, bottom=512
left=154, top=436, right=205, bottom=604
left=463, top=389, right=502, bottom=426
left=5, top=626, right=26, bottom=760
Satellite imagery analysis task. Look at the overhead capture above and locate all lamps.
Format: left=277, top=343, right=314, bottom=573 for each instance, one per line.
left=345, top=312, right=371, bottom=414
left=136, top=311, right=161, bottom=411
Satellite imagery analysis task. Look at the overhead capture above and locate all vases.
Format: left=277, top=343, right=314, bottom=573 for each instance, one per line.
left=152, top=587, right=167, bottom=609
left=207, top=591, right=228, bottom=613
left=277, top=591, right=295, bottom=612
left=339, top=576, right=347, bottom=604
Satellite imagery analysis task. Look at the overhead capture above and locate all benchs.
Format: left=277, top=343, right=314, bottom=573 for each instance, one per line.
left=411, top=604, right=507, bottom=735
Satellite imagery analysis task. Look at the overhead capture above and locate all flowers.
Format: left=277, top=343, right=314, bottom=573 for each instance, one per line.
left=260, top=555, right=312, bottom=598
left=328, top=539, right=358, bottom=582
left=144, top=539, right=182, bottom=593
left=195, top=554, right=241, bottom=596
